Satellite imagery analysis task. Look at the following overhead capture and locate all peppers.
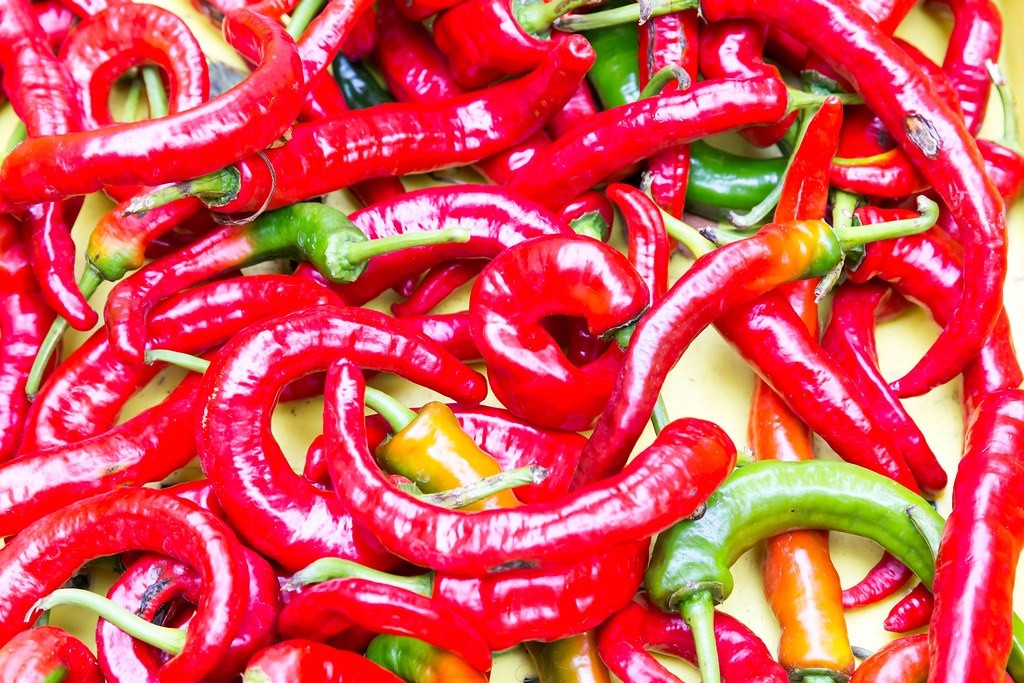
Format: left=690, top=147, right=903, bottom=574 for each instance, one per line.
left=0, top=0, right=1024, bottom=683
